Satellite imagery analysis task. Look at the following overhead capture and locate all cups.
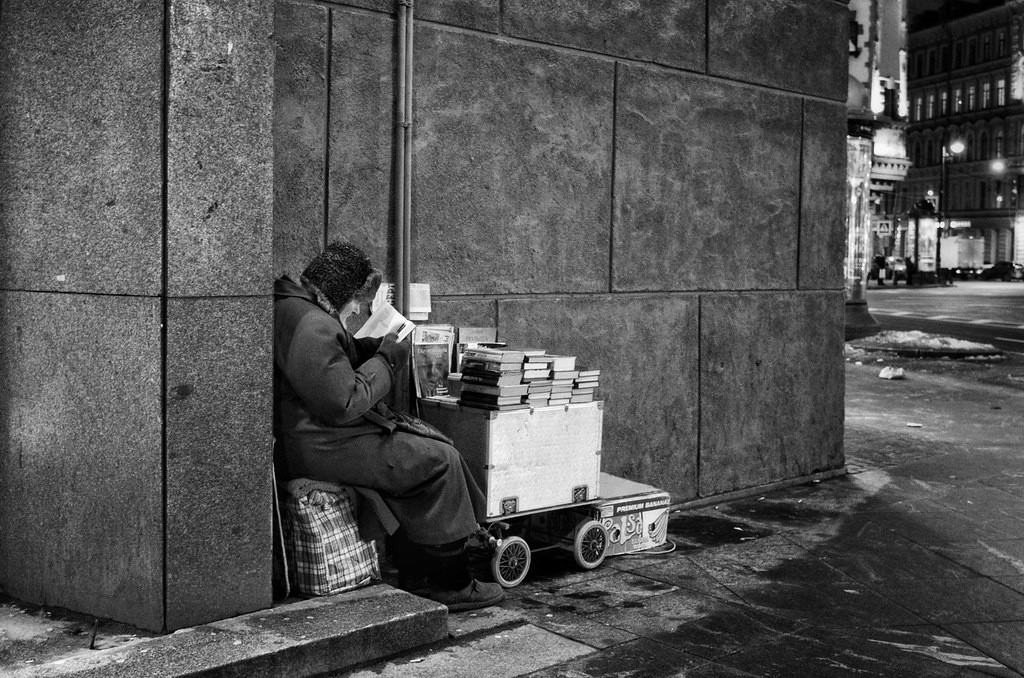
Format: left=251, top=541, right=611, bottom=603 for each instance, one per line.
left=447, top=372, right=463, bottom=398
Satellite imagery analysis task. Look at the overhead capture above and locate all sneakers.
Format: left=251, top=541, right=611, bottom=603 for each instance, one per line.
left=398, top=571, right=430, bottom=591
left=431, top=579, right=504, bottom=613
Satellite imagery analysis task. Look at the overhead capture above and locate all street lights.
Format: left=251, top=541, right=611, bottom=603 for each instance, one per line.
left=936, top=140, right=965, bottom=284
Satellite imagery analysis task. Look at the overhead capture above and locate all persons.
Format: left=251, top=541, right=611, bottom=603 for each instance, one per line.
left=273, top=243, right=503, bottom=610
left=874, top=252, right=886, bottom=285
left=905, top=257, right=914, bottom=285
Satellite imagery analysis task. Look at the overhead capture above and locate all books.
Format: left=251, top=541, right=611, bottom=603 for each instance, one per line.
left=352, top=302, right=416, bottom=343
left=413, top=325, right=600, bottom=411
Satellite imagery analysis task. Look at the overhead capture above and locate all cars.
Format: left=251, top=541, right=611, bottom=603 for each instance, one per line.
left=980, top=261, right=1024, bottom=282
left=878, top=254, right=982, bottom=286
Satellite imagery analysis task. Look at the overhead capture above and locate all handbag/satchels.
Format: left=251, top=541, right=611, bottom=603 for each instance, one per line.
left=280, top=490, right=384, bottom=596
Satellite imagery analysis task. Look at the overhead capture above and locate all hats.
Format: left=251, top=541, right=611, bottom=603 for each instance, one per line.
left=300, top=241, right=382, bottom=318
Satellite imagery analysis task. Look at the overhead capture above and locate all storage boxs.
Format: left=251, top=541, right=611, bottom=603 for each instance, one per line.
left=532, top=472, right=671, bottom=557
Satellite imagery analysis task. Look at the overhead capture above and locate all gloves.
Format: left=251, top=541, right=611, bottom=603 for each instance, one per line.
left=375, top=332, right=411, bottom=372
